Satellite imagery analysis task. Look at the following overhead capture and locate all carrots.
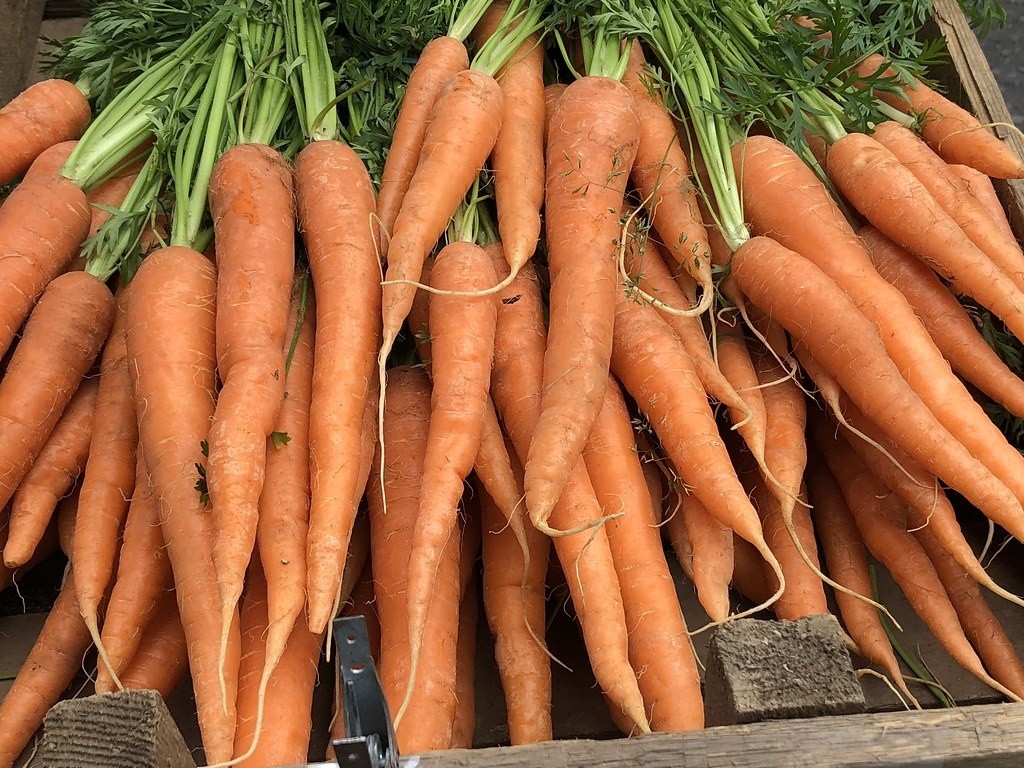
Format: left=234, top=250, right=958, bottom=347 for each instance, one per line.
left=0, top=0, right=1024, bottom=768
